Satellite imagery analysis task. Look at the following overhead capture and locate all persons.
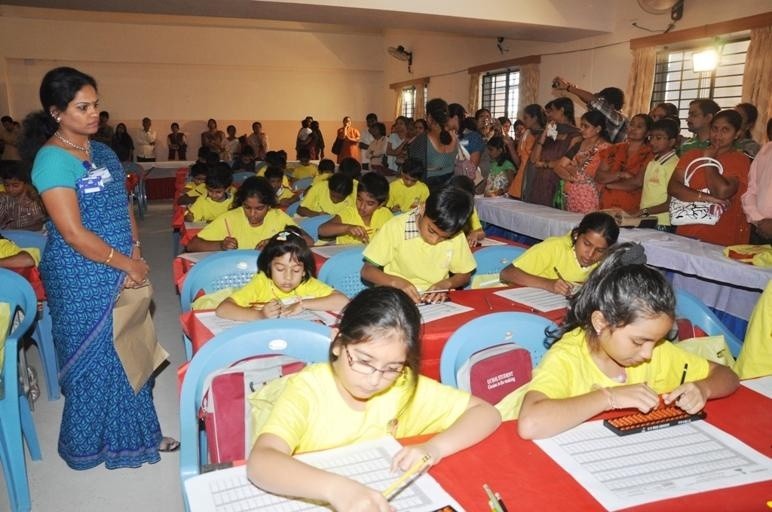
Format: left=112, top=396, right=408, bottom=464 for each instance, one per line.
left=517, top=241, right=739, bottom=441
left=297, top=173, right=357, bottom=218
left=514, top=119, right=525, bottom=150
left=424, top=98, right=459, bottom=188
left=360, top=186, right=474, bottom=304
left=178, top=163, right=239, bottom=206
left=256, top=149, right=287, bottom=178
left=387, top=116, right=409, bottom=174
left=741, top=118, right=772, bottom=249
left=364, top=123, right=388, bottom=169
left=337, top=117, right=361, bottom=167
left=1, top=114, right=21, bottom=172
left=396, top=119, right=428, bottom=177
left=184, top=166, right=241, bottom=224
left=444, top=175, right=486, bottom=247
left=357, top=113, right=378, bottom=171
left=295, top=116, right=315, bottom=162
left=552, top=76, right=625, bottom=143
left=595, top=114, right=656, bottom=220
left=1, top=229, right=40, bottom=402
left=391, top=124, right=396, bottom=135
left=645, top=102, right=692, bottom=152
left=265, top=151, right=290, bottom=188
left=338, top=157, right=360, bottom=189
left=475, top=108, right=495, bottom=184
left=510, top=105, right=547, bottom=201
left=242, top=122, right=268, bottom=161
left=499, top=212, right=620, bottom=297
left=301, top=121, right=325, bottom=160
left=203, top=153, right=222, bottom=172
left=384, top=157, right=431, bottom=212
left=181, top=164, right=208, bottom=195
left=447, top=103, right=483, bottom=181
left=498, top=117, right=521, bottom=170
left=166, top=123, right=186, bottom=161
left=111, top=123, right=134, bottom=164
left=529, top=97, right=583, bottom=209
left=735, top=277, right=771, bottom=380
left=627, top=119, right=681, bottom=234
left=484, top=136, right=516, bottom=199
left=215, top=231, right=352, bottom=321
left=676, top=98, right=721, bottom=160
left=32, top=67, right=180, bottom=470
left=89, top=111, right=113, bottom=149
left=318, top=172, right=394, bottom=246
left=133, top=118, right=156, bottom=162
left=221, top=125, right=240, bottom=162
left=264, top=166, right=300, bottom=206
left=298, top=120, right=316, bottom=159
left=1, top=161, right=46, bottom=231
left=303, top=159, right=335, bottom=197
left=555, top=111, right=612, bottom=215
left=246, top=285, right=501, bottom=512
left=186, top=177, right=314, bottom=252
left=290, top=149, right=317, bottom=182
left=667, top=110, right=752, bottom=247
left=463, top=115, right=476, bottom=131
left=731, top=103, right=762, bottom=163
left=201, top=118, right=224, bottom=160
left=544, top=100, right=555, bottom=125
left=190, top=146, right=211, bottom=178
left=231, top=145, right=257, bottom=174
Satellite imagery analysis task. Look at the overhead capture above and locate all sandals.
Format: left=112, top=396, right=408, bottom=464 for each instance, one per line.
left=26, top=366, right=40, bottom=402
left=159, top=437, right=179, bottom=451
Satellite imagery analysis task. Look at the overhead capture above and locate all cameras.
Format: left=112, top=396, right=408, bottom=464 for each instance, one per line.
left=549, top=81, right=561, bottom=88
left=483, top=118, right=499, bottom=129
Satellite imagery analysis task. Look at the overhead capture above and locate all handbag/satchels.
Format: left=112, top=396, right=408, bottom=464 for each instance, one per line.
left=332, top=134, right=343, bottom=155
left=669, top=158, right=729, bottom=228
left=454, top=142, right=477, bottom=182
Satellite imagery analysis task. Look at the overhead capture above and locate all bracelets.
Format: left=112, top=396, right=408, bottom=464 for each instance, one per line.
left=601, top=386, right=618, bottom=410
left=642, top=207, right=649, bottom=216
left=543, top=159, right=549, bottom=169
left=104, top=248, right=114, bottom=265
left=536, top=141, right=543, bottom=146
left=567, top=83, right=571, bottom=92
left=131, top=239, right=143, bottom=246
left=500, top=188, right=505, bottom=195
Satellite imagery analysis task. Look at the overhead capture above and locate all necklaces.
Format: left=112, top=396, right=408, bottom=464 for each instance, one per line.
left=55, top=130, right=91, bottom=151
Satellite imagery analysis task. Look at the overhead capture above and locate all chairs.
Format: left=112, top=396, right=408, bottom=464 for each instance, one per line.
left=0, top=267, right=42, bottom=512
left=179, top=248, right=266, bottom=361
left=177, top=316, right=339, bottom=510
left=674, top=284, right=745, bottom=360
left=301, top=213, right=333, bottom=244
left=0, top=228, right=71, bottom=401
left=438, top=310, right=565, bottom=391
left=464, top=246, right=527, bottom=291
left=318, top=247, right=372, bottom=300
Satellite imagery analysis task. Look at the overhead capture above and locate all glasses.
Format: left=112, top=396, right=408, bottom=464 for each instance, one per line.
left=343, top=342, right=407, bottom=381
left=647, top=135, right=674, bottom=142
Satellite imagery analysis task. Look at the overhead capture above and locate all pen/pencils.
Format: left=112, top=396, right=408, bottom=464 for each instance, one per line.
left=483, top=483, right=509, bottom=512
left=554, top=266, right=566, bottom=283
left=674, top=364, right=690, bottom=404
left=194, top=189, right=203, bottom=196
left=186, top=205, right=191, bottom=213
left=383, top=454, right=429, bottom=499
left=425, top=288, right=456, bottom=293
left=249, top=301, right=268, bottom=305
left=317, top=202, right=324, bottom=212
left=224, top=218, right=231, bottom=238
left=483, top=297, right=492, bottom=309
